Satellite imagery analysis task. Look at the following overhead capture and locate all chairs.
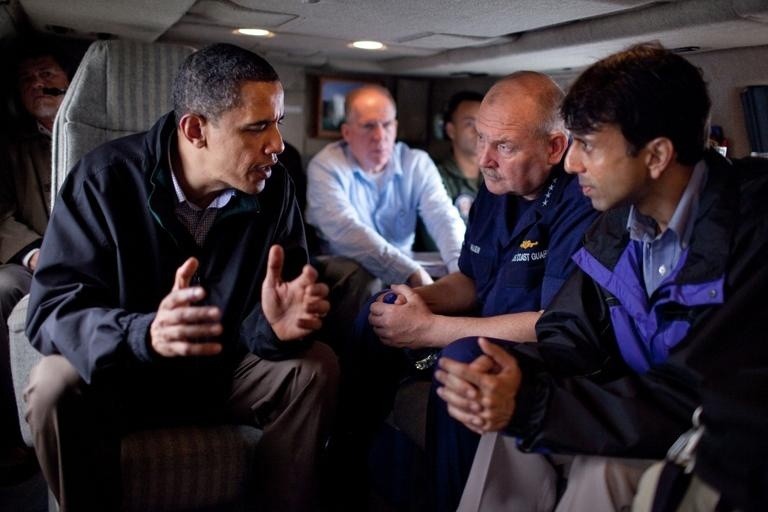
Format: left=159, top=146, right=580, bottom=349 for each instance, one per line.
left=8, top=34, right=272, bottom=506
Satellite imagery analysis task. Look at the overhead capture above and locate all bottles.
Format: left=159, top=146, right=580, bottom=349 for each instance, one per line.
left=384, top=293, right=442, bottom=380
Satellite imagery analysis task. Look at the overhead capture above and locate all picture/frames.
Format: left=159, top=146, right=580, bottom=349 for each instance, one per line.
left=314, top=76, right=368, bottom=139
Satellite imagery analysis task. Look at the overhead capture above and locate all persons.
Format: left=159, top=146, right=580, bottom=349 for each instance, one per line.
left=435, top=41, right=768, bottom=511
left=305, top=84, right=466, bottom=320
left=335, top=72, right=597, bottom=511
left=413, top=90, right=484, bottom=253
left=0, top=49, right=72, bottom=322
left=24, top=43, right=340, bottom=512
